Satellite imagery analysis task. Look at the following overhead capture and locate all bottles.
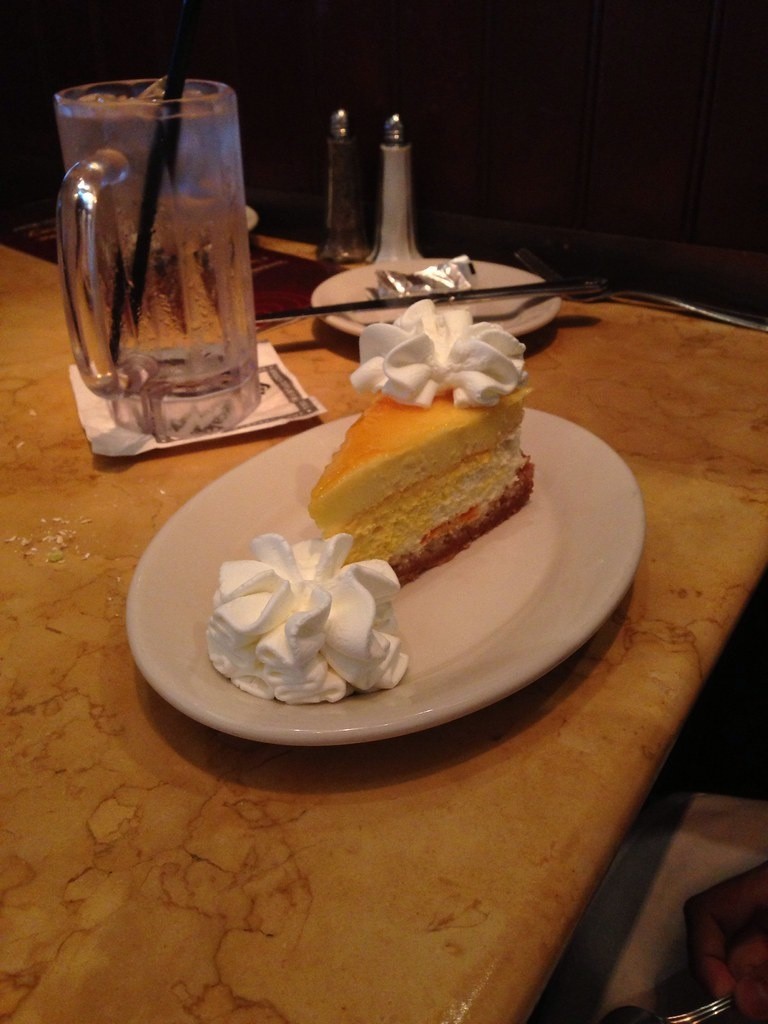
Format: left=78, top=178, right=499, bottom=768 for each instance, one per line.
left=313, top=108, right=371, bottom=261
left=371, top=112, right=424, bottom=263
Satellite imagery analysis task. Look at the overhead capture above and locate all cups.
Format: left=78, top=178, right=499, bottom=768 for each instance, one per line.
left=52, top=77, right=263, bottom=442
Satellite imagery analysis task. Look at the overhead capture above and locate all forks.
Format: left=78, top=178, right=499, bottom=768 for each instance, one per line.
left=514, top=247, right=768, bottom=334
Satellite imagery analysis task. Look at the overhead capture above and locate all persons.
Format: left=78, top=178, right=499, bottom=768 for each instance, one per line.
left=683, top=858, right=768, bottom=1024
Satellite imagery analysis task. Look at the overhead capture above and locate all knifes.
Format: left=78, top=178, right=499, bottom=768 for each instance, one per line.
left=256, top=279, right=606, bottom=324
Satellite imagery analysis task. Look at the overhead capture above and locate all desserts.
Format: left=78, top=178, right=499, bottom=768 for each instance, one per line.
left=206, top=298, right=535, bottom=705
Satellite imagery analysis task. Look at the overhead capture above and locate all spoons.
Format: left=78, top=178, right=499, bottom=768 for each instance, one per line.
left=597, top=992, right=735, bottom=1024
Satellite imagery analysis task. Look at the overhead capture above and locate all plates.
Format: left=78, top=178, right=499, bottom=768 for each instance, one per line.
left=311, top=259, right=563, bottom=338
left=123, top=407, right=644, bottom=746
